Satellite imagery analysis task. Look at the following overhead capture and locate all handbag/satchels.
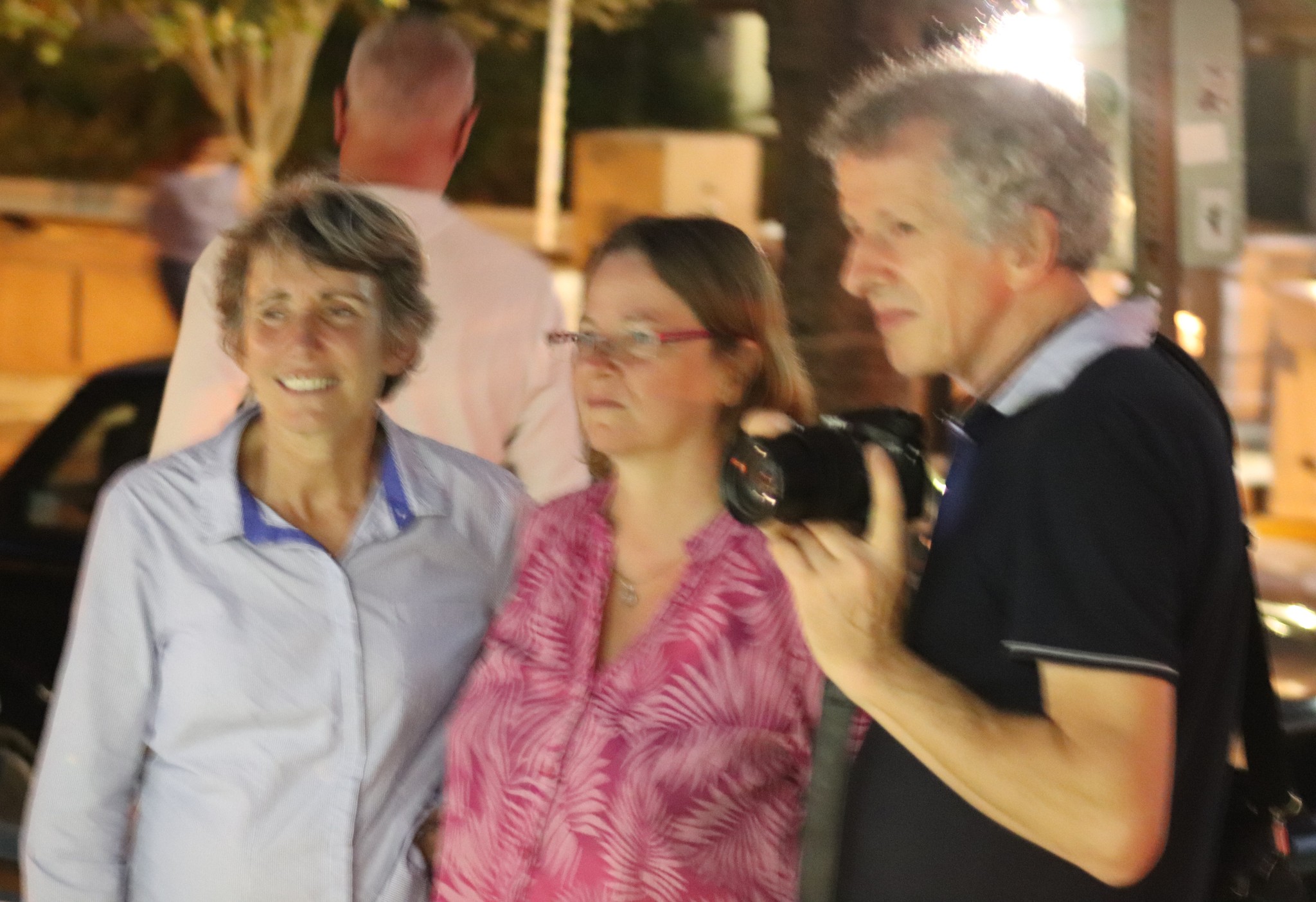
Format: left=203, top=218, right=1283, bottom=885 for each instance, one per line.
left=1214, top=758, right=1308, bottom=902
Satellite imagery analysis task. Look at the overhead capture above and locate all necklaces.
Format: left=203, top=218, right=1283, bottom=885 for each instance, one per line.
left=608, top=497, right=720, bottom=608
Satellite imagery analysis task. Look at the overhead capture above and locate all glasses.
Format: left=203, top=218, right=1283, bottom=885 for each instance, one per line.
left=545, top=328, right=716, bottom=369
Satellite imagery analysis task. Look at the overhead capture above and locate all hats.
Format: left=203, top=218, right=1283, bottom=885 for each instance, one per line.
left=148, top=166, right=254, bottom=261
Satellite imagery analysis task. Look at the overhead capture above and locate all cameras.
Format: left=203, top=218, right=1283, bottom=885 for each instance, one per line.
left=717, top=401, right=928, bottom=527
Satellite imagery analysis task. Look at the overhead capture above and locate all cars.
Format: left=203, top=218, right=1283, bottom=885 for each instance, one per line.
left=2, top=352, right=171, bottom=688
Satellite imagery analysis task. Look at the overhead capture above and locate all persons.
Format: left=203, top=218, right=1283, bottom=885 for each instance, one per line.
left=150, top=13, right=600, bottom=527
left=433, top=205, right=910, bottom=902
left=13, top=166, right=543, bottom=900
left=740, top=60, right=1287, bottom=902
